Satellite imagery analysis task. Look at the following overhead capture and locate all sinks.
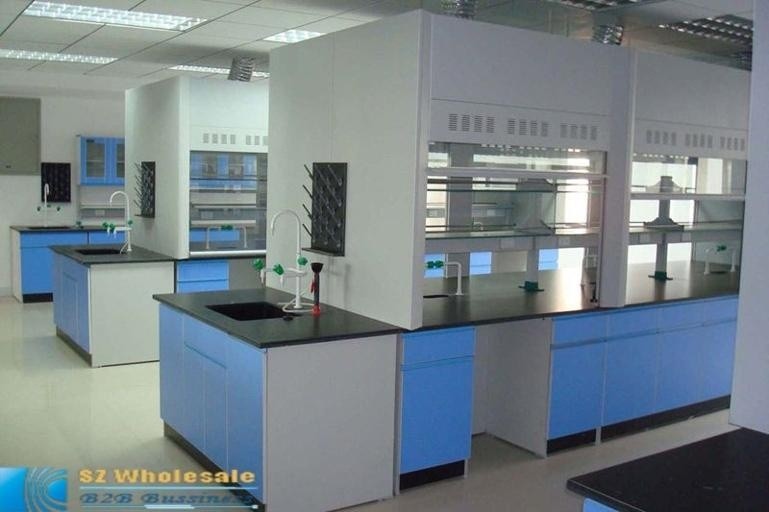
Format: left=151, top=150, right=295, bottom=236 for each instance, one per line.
left=423, top=295, right=448, bottom=298
left=28, top=226, right=70, bottom=229
left=74, top=249, right=127, bottom=255
left=205, top=301, right=302, bottom=322
left=710, top=270, right=728, bottom=274
left=218, top=246, right=237, bottom=249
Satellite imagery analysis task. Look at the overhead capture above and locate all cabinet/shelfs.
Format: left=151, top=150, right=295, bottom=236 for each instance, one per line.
left=395, top=329, right=473, bottom=495
left=428, top=139, right=749, bottom=254
left=78, top=135, right=129, bottom=185
left=146, top=304, right=396, bottom=512
left=8, top=232, right=129, bottom=304
left=485, top=299, right=739, bottom=460
left=53, top=253, right=176, bottom=371
left=188, top=151, right=269, bottom=258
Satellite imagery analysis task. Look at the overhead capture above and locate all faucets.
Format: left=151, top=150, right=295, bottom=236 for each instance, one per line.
left=206, top=225, right=226, bottom=250
left=721, top=245, right=738, bottom=272
left=703, top=244, right=721, bottom=275
left=434, top=260, right=463, bottom=297
left=424, top=261, right=433, bottom=270
left=226, top=225, right=247, bottom=250
left=268, top=210, right=306, bottom=308
left=44, top=183, right=50, bottom=227
left=109, top=190, right=132, bottom=252
